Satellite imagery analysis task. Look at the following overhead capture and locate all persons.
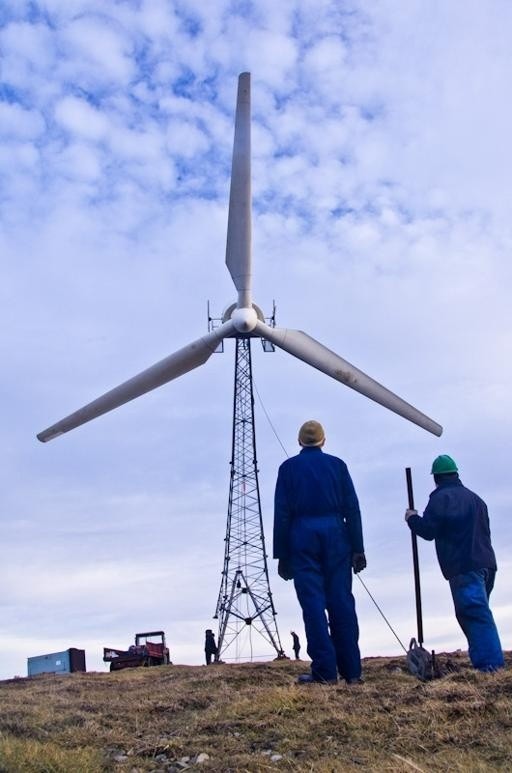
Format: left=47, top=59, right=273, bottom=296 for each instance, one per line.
left=405, top=454, right=505, bottom=672
left=205, top=630, right=218, bottom=666
left=290, top=632, right=301, bottom=660
left=273, top=421, right=366, bottom=683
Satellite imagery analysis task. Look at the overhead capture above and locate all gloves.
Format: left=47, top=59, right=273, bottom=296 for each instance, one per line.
left=352, top=552, right=366, bottom=574
left=405, top=509, right=417, bottom=521
left=278, top=558, right=295, bottom=581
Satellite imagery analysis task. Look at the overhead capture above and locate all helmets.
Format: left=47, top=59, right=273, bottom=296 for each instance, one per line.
left=299, top=421, right=325, bottom=446
left=430, top=455, right=458, bottom=474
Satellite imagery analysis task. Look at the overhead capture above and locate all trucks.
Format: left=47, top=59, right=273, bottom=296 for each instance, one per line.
left=103, top=631, right=171, bottom=673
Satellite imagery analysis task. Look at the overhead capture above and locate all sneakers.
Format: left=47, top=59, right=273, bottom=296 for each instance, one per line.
left=299, top=674, right=337, bottom=684
left=347, top=674, right=365, bottom=684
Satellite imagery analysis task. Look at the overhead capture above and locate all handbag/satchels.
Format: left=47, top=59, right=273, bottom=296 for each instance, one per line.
left=407, top=638, right=440, bottom=681
